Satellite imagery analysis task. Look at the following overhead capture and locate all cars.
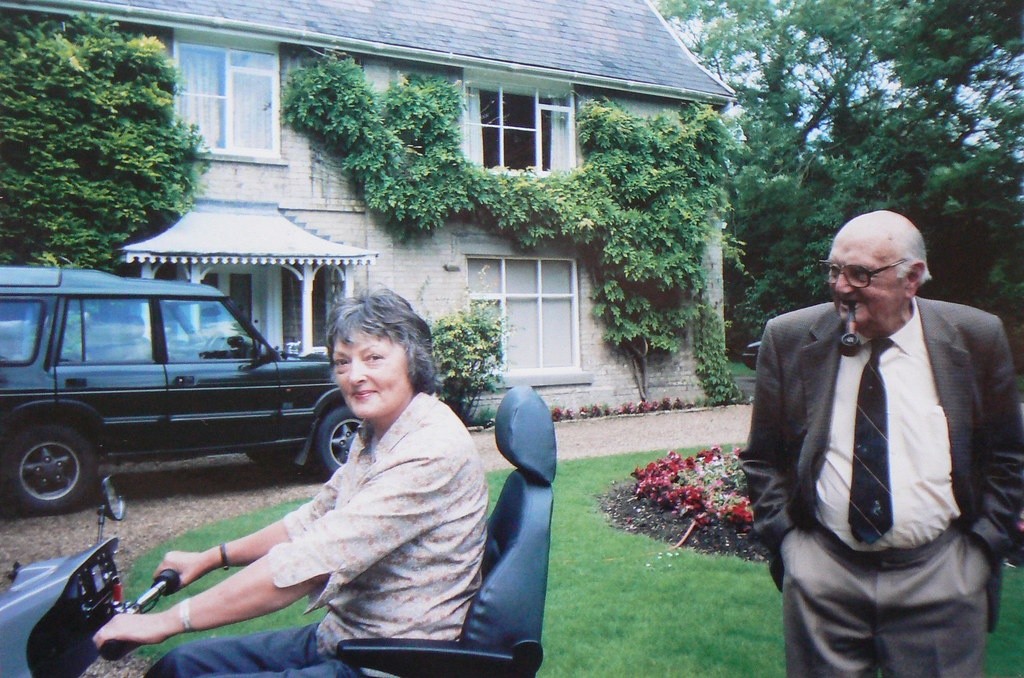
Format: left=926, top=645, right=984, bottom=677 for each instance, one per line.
left=740, top=340, right=762, bottom=370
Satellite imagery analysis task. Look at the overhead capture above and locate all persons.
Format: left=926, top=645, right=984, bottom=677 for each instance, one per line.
left=738, top=210, right=1023, bottom=678
left=91, top=287, right=489, bottom=678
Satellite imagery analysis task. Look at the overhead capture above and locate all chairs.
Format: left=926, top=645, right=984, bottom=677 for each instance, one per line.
left=118, top=314, right=154, bottom=362
left=88, top=309, right=115, bottom=363
left=336, top=386, right=557, bottom=677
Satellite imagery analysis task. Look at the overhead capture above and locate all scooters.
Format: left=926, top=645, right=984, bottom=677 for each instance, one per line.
left=0, top=375, right=559, bottom=677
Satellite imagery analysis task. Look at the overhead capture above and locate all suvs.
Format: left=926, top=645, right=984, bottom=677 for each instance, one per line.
left=0, top=262, right=376, bottom=517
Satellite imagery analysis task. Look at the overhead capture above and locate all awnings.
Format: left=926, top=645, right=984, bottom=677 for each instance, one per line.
left=119, top=202, right=380, bottom=268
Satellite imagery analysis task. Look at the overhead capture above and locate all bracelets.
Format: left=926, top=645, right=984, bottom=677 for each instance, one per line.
left=180, top=599, right=191, bottom=631
left=219, top=543, right=227, bottom=565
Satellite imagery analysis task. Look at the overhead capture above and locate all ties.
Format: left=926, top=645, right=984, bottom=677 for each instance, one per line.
left=848, top=340, right=895, bottom=547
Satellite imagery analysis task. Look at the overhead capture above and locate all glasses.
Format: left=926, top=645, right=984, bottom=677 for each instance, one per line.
left=817, top=259, right=907, bottom=290
